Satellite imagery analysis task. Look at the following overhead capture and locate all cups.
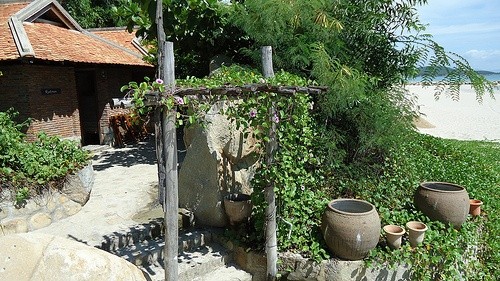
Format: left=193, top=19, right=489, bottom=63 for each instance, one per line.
left=383, top=225, right=405, bottom=250
left=406, top=221, right=428, bottom=247
left=470, top=199, right=483, bottom=217
